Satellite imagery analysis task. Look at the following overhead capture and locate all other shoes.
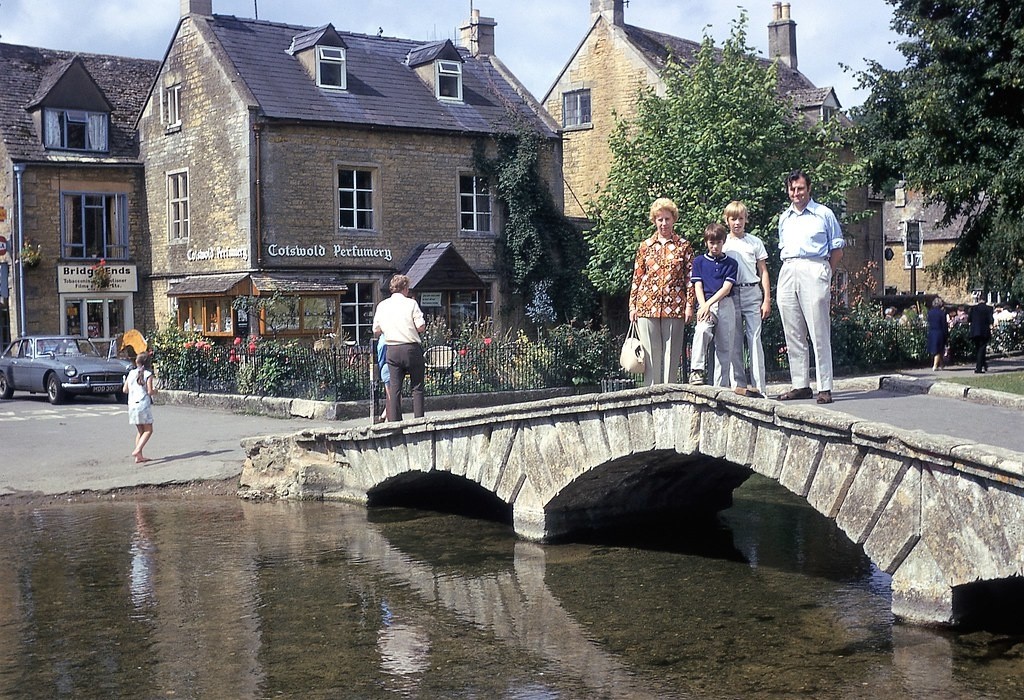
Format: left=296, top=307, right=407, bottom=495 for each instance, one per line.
left=932, top=366, right=942, bottom=371
left=689, top=371, right=704, bottom=385
left=777, top=386, right=813, bottom=400
left=817, top=390, right=831, bottom=404
left=984, top=366, right=988, bottom=371
left=975, top=371, right=985, bottom=373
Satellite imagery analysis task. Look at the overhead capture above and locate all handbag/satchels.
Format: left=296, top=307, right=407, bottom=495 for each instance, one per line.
left=619, top=320, right=645, bottom=373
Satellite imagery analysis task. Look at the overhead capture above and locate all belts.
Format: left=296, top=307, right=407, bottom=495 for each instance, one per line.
left=738, top=283, right=758, bottom=287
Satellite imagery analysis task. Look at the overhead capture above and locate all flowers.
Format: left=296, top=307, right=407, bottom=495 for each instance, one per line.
left=16, top=236, right=42, bottom=267
left=92, top=259, right=111, bottom=290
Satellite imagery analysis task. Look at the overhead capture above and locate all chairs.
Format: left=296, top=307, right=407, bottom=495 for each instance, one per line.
left=424, top=345, right=458, bottom=369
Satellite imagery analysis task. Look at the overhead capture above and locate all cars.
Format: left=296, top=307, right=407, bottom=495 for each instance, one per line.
left=0, top=335, right=129, bottom=405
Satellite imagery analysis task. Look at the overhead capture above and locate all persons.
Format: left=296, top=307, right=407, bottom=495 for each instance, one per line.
left=993, top=303, right=1017, bottom=327
left=371, top=275, right=425, bottom=421
left=375, top=331, right=402, bottom=405
left=628, top=197, right=695, bottom=386
left=885, top=305, right=923, bottom=326
left=689, top=223, right=739, bottom=386
left=926, top=298, right=948, bottom=371
left=122, top=351, right=158, bottom=463
left=966, top=293, right=994, bottom=373
left=722, top=201, right=771, bottom=399
left=775, top=167, right=844, bottom=403
left=946, top=305, right=969, bottom=325
